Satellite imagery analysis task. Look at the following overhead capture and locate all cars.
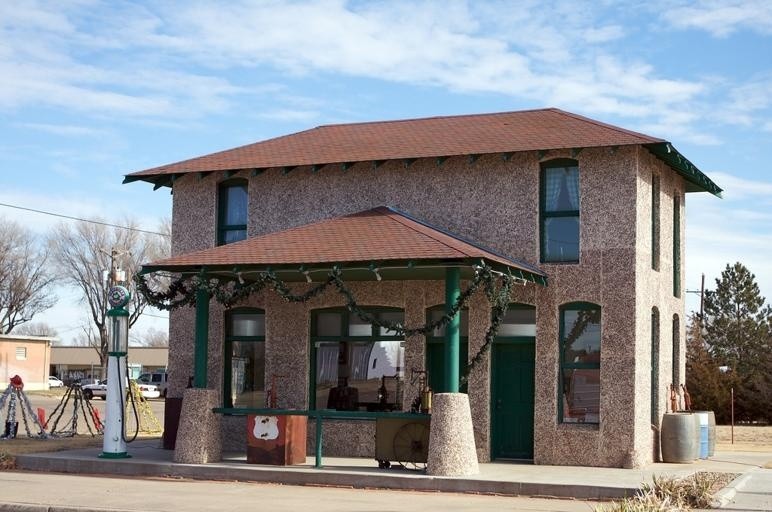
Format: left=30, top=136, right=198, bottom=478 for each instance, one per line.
left=48, top=375, right=66, bottom=388
left=70, top=377, right=84, bottom=389
left=80, top=377, right=160, bottom=402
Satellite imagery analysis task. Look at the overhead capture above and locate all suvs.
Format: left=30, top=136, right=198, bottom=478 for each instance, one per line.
left=138, top=367, right=168, bottom=400
